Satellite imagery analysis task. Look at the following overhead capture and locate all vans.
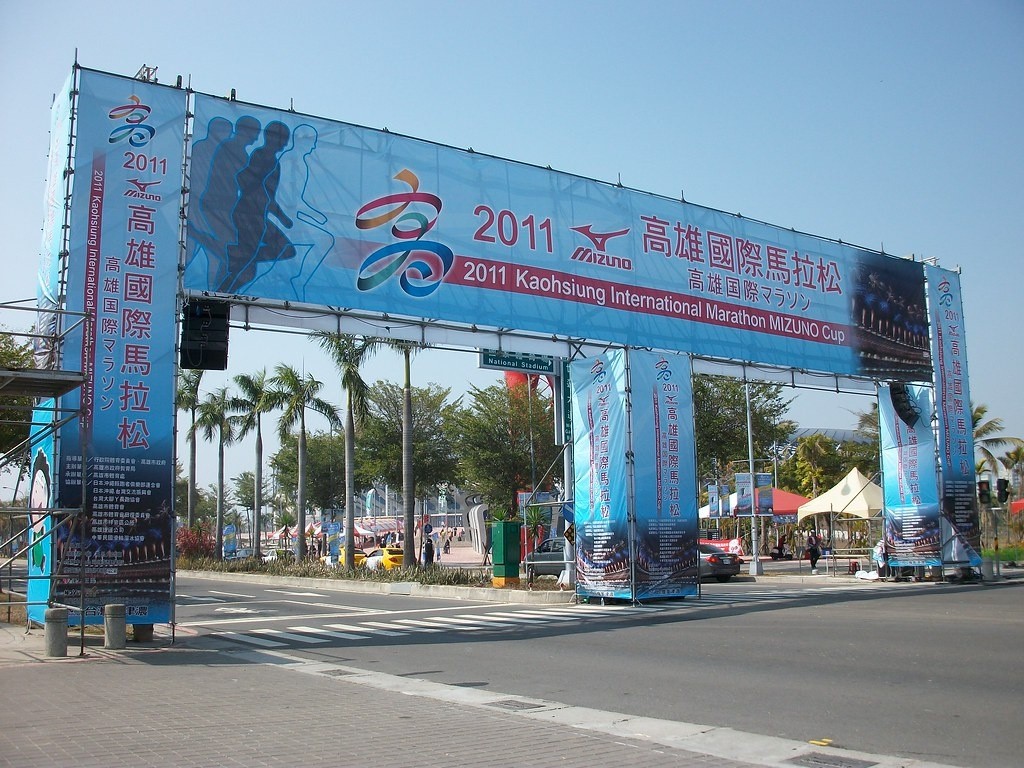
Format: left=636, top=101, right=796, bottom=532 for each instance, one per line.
left=523, top=538, right=566, bottom=580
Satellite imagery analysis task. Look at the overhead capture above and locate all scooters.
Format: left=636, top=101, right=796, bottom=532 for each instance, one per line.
left=772, top=543, right=792, bottom=561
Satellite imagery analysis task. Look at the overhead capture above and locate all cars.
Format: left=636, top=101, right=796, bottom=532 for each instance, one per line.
left=320, top=548, right=367, bottom=567
left=260, top=549, right=295, bottom=564
left=358, top=547, right=417, bottom=572
left=226, top=548, right=265, bottom=561
left=697, top=544, right=741, bottom=583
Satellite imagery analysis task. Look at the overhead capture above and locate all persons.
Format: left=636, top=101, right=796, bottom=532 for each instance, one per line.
left=318, top=542, right=321, bottom=558
left=422, top=526, right=465, bottom=563
left=852, top=271, right=928, bottom=350
left=778, top=536, right=787, bottom=550
left=376, top=529, right=401, bottom=548
left=807, top=529, right=822, bottom=569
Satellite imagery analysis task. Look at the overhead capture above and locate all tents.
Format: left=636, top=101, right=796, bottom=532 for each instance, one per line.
left=699, top=492, right=738, bottom=517
left=314, top=524, right=376, bottom=551
left=734, top=486, right=812, bottom=539
left=358, top=519, right=402, bottom=536
left=1011, top=498, right=1024, bottom=515
left=798, top=467, right=882, bottom=574
left=268, top=523, right=317, bottom=544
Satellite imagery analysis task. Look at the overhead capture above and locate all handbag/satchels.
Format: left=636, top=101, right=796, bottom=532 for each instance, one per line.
left=805, top=537, right=811, bottom=550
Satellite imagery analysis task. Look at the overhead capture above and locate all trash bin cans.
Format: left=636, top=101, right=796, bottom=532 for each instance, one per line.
left=822, top=547, right=832, bottom=559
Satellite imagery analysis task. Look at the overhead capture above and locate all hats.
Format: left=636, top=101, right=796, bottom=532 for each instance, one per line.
left=811, top=530, right=815, bottom=533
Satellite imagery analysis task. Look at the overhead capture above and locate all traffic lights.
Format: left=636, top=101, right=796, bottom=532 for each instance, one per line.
left=977, top=480, right=990, bottom=503
left=996, top=479, right=1010, bottom=503
